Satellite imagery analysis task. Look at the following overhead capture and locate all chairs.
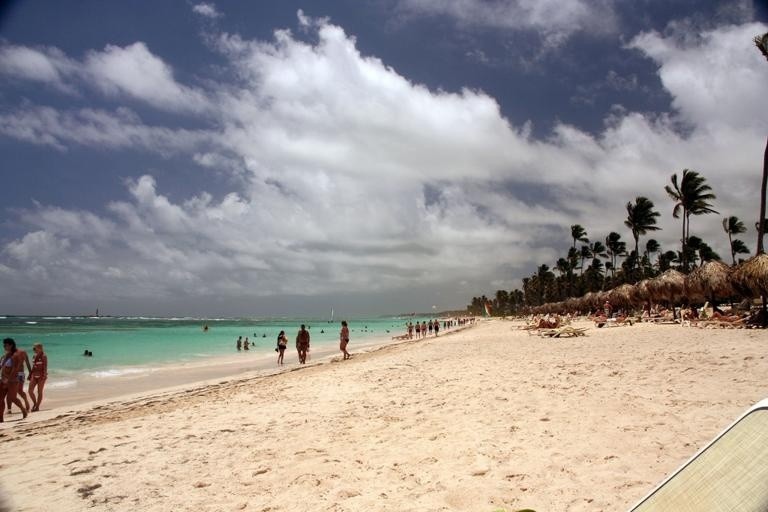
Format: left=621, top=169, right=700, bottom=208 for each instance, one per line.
left=525, top=310, right=761, bottom=339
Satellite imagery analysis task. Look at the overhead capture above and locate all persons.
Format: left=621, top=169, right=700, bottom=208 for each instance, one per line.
left=236, top=336, right=242, bottom=350
left=6, top=345, right=32, bottom=416
left=244, top=337, right=250, bottom=350
left=295, top=323, right=310, bottom=364
left=338, top=319, right=350, bottom=360
left=276, top=330, right=287, bottom=364
left=498, top=298, right=757, bottom=339
left=405, top=314, right=477, bottom=340
left=0, top=337, right=28, bottom=423
left=26, top=340, right=48, bottom=412
